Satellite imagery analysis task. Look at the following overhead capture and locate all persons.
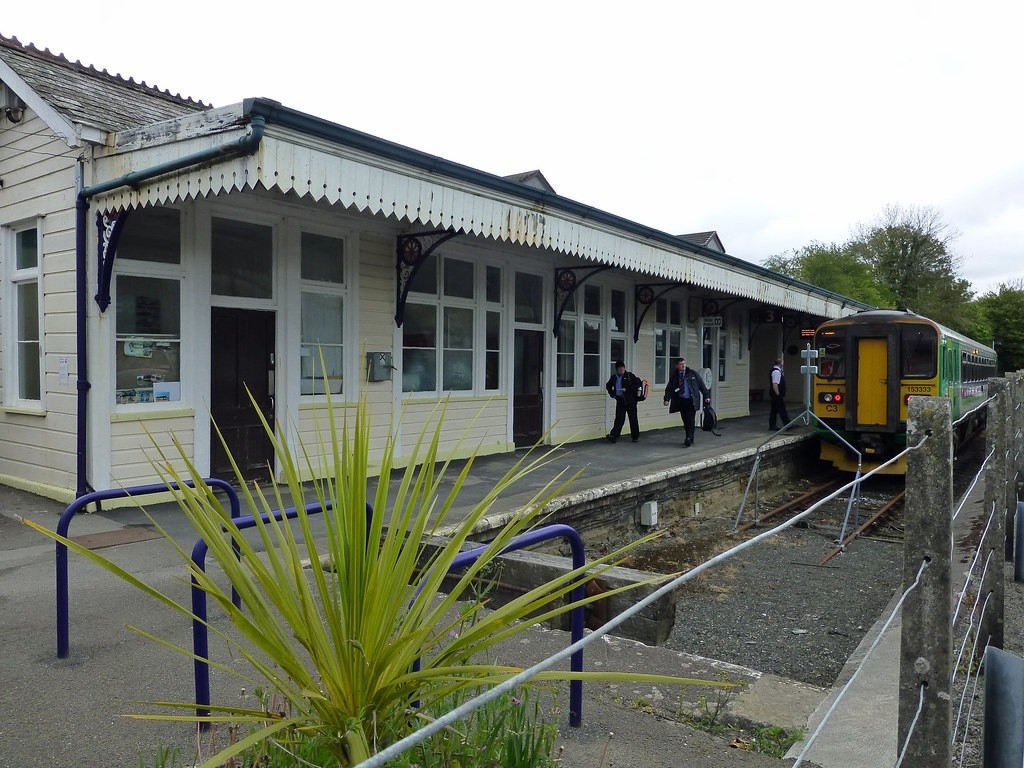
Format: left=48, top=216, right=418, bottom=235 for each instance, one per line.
left=768, top=358, right=800, bottom=431
left=664, top=358, right=711, bottom=447
left=606, top=361, right=639, bottom=444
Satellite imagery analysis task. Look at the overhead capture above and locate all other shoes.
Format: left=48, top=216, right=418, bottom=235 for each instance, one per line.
left=787, top=424, right=800, bottom=429
left=632, top=437, right=638, bottom=442
left=769, top=425, right=781, bottom=431
left=685, top=437, right=691, bottom=447
left=606, top=434, right=616, bottom=443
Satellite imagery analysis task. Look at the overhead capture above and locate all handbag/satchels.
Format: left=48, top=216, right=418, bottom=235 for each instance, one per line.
left=700, top=400, right=721, bottom=437
left=635, top=379, right=649, bottom=401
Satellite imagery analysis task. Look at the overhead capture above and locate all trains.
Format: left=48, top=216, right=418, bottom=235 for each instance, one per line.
left=808, top=306, right=997, bottom=481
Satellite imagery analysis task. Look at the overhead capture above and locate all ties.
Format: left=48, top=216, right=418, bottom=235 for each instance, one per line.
left=678, top=372, right=685, bottom=395
